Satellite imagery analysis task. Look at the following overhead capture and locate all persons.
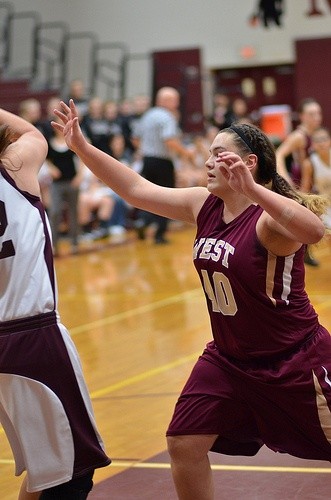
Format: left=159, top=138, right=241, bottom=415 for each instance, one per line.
left=0, top=107, right=112, bottom=500
left=18, top=88, right=331, bottom=268
left=50, top=99, right=331, bottom=500
